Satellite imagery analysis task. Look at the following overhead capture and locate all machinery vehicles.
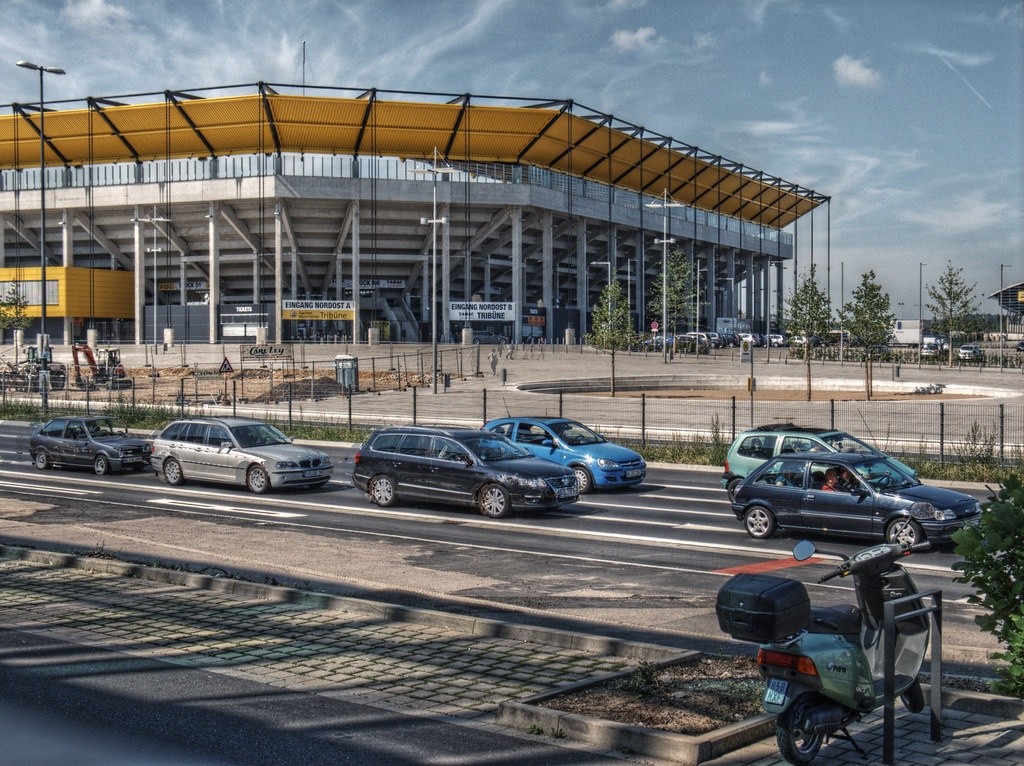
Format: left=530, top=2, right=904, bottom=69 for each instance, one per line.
left=70, top=342, right=133, bottom=393
left=1, top=346, right=66, bottom=392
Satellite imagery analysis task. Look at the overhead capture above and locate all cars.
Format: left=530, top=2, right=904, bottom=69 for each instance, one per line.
left=921, top=342, right=951, bottom=360
left=868, top=345, right=890, bottom=360
left=640, top=335, right=673, bottom=353
left=1016, top=342, right=1024, bottom=353
left=711, top=332, right=740, bottom=349
left=958, top=345, right=981, bottom=360
left=675, top=335, right=706, bottom=354
left=479, top=416, right=647, bottom=496
left=686, top=332, right=712, bottom=348
left=472, top=330, right=509, bottom=345
left=720, top=423, right=919, bottom=505
left=760, top=332, right=829, bottom=349
left=731, top=450, right=983, bottom=552
left=739, top=333, right=759, bottom=347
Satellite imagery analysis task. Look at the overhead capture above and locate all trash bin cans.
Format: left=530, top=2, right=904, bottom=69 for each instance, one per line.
left=334, top=354, right=359, bottom=392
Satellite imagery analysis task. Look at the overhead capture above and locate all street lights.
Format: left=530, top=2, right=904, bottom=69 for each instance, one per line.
left=591, top=261, right=612, bottom=338
left=14, top=59, right=67, bottom=360
left=406, top=146, right=461, bottom=394
left=999, top=263, right=1013, bottom=374
left=129, top=205, right=174, bottom=355
left=696, top=259, right=704, bottom=359
left=644, top=187, right=686, bottom=362
left=918, top=263, right=928, bottom=370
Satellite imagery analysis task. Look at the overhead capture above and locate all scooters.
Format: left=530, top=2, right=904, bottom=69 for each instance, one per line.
left=713, top=502, right=938, bottom=765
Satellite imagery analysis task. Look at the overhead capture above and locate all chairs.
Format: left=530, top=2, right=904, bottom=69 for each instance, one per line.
left=495, top=427, right=505, bottom=435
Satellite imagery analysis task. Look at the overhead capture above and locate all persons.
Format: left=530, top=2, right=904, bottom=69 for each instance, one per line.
left=823, top=468, right=840, bottom=492
left=774, top=464, right=802, bottom=487
left=841, top=466, right=891, bottom=494
left=487, top=348, right=499, bottom=375
left=808, top=440, right=841, bottom=454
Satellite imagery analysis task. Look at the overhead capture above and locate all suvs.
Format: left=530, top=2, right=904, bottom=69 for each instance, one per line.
left=30, top=415, right=152, bottom=476
left=351, top=425, right=582, bottom=519
left=150, top=416, right=335, bottom=493
